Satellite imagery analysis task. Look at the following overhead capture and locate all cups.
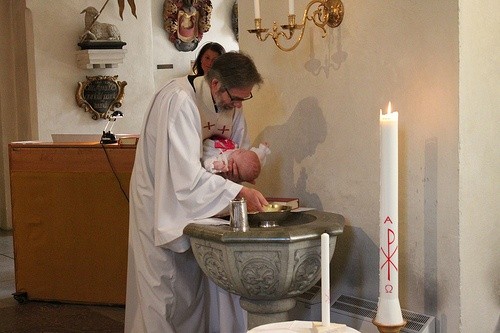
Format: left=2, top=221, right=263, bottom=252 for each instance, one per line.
left=229, top=199, right=249, bottom=232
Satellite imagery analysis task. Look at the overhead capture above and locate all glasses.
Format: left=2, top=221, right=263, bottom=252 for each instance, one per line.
left=224, top=88, right=253, bottom=101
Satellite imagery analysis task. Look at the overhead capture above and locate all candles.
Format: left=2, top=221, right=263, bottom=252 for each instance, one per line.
left=321, top=230, right=330, bottom=327
left=254, top=0, right=261, bottom=19
left=375, top=100, right=405, bottom=326
left=289, top=0, right=295, bottom=15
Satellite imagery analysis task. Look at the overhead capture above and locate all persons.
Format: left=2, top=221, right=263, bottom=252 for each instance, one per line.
left=124, top=50, right=269, bottom=333
left=201, top=135, right=271, bottom=181
left=193, top=43, right=227, bottom=76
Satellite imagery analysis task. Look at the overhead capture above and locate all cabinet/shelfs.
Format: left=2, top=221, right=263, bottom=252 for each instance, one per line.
left=8, top=141, right=136, bottom=306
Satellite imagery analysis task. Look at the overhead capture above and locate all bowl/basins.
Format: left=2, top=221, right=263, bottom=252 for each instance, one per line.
left=247, top=204, right=294, bottom=222
